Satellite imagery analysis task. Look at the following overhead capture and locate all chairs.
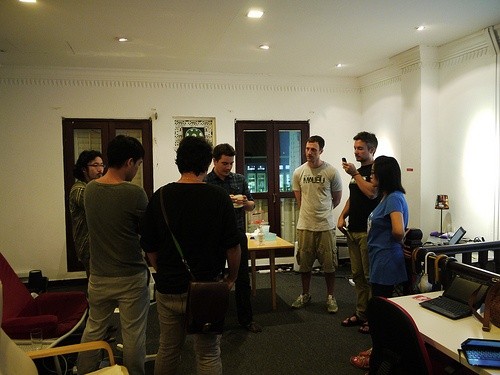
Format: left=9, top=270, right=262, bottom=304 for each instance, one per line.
left=0, top=326, right=129, bottom=375
left=0, top=251, right=88, bottom=338
left=368, top=296, right=455, bottom=375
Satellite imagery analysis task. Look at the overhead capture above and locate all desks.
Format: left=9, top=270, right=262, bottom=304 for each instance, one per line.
left=387, top=290, right=500, bottom=375
left=245, top=233, right=294, bottom=312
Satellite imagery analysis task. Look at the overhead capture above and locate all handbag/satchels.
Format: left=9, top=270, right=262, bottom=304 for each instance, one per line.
left=470, top=277, right=500, bottom=332
left=183, top=281, right=229, bottom=335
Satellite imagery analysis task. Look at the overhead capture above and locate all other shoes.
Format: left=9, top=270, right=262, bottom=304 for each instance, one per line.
left=241, top=320, right=263, bottom=332
left=349, top=355, right=370, bottom=369
left=359, top=348, right=372, bottom=356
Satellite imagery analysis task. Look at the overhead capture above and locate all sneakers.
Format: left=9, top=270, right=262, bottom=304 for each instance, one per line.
left=326, top=295, right=338, bottom=313
left=292, top=293, right=311, bottom=309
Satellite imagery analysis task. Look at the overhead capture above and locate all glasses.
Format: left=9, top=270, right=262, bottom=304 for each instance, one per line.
left=87, top=163, right=104, bottom=168
left=371, top=170, right=375, bottom=177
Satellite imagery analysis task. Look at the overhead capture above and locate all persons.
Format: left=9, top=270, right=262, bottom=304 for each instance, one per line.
left=141, top=136, right=241, bottom=375
left=337, top=131, right=378, bottom=333
left=351, top=155, right=408, bottom=369
left=68, top=150, right=114, bottom=299
left=292, top=136, right=342, bottom=312
left=76, top=135, right=148, bottom=375
left=204, top=144, right=262, bottom=334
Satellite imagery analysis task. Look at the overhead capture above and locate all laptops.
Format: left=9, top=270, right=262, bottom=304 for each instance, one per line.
left=461, top=338, right=500, bottom=367
left=448, top=227, right=466, bottom=245
left=419, top=273, right=491, bottom=319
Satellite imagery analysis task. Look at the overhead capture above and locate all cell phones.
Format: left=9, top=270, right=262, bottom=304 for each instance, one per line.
left=342, top=158, right=346, bottom=166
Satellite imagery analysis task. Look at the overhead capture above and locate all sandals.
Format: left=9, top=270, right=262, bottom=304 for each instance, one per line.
left=359, top=324, right=370, bottom=334
left=342, top=313, right=363, bottom=326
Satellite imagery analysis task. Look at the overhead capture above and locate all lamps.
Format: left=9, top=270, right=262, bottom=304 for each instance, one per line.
left=434, top=195, right=449, bottom=237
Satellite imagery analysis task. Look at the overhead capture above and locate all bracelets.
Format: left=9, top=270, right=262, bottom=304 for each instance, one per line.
left=351, top=173, right=359, bottom=180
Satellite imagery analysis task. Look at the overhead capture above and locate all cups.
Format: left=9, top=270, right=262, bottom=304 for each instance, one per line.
left=262, top=224, right=270, bottom=234
left=258, top=233, right=263, bottom=243
left=29, top=270, right=42, bottom=291
left=266, top=233, right=275, bottom=240
